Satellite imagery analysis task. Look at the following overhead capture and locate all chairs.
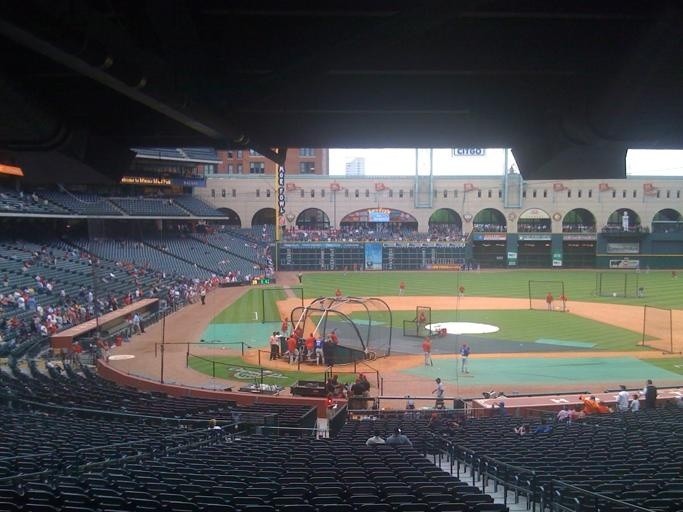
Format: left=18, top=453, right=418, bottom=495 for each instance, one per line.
left=1, top=356, right=393, bottom=511
left=386, top=400, right=682, bottom=512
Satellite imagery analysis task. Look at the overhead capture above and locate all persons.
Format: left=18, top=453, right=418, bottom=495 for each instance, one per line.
left=139, top=189, right=182, bottom=206
left=1, top=187, right=49, bottom=212
